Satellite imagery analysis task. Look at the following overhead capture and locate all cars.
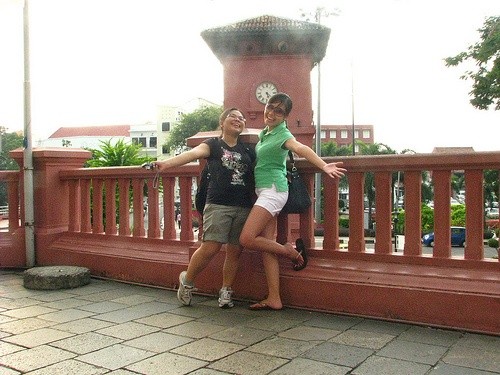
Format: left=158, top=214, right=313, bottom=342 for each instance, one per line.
left=424, top=188, right=499, bottom=219
left=127, top=145, right=196, bottom=214
left=423, top=225, right=467, bottom=248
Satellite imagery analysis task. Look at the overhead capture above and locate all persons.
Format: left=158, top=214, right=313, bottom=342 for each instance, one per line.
left=238, top=92, right=348, bottom=309
left=147, top=107, right=255, bottom=310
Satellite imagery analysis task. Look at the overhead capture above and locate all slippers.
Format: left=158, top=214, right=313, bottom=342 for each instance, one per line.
left=293, top=238, right=308, bottom=272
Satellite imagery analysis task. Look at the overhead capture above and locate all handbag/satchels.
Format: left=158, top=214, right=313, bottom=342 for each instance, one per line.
left=281, top=152, right=312, bottom=216
left=195, top=164, right=209, bottom=216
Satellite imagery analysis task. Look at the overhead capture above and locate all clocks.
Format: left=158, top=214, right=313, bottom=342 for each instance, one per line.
left=255, top=81, right=278, bottom=105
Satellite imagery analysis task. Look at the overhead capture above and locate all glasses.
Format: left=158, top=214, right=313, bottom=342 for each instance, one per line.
left=266, top=104, right=285, bottom=117
left=226, top=114, right=246, bottom=122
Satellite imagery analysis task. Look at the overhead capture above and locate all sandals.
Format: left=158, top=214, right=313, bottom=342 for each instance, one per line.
left=250, top=298, right=283, bottom=311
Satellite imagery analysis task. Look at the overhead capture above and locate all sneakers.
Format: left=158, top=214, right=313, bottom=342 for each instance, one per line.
left=217, top=287, right=234, bottom=309
left=177, top=271, right=193, bottom=306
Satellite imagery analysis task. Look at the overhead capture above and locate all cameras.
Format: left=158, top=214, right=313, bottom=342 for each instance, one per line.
left=141, top=162, right=159, bottom=170
